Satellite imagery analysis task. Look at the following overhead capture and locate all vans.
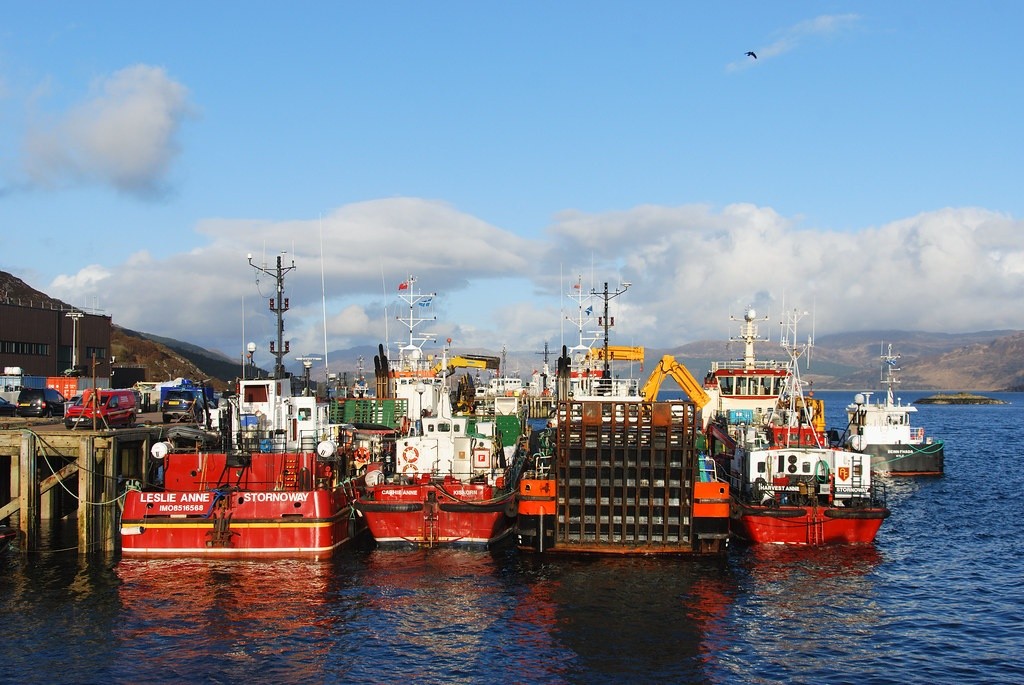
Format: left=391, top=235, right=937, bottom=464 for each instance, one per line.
left=65, top=389, right=137, bottom=430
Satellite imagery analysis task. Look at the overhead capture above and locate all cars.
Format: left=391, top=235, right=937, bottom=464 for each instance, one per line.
left=16, top=387, right=69, bottom=418
left=162, top=390, right=216, bottom=423
left=0, top=397, right=17, bottom=417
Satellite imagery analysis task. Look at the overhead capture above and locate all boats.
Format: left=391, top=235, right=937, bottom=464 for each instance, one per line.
left=514, top=252, right=731, bottom=558
left=354, top=274, right=531, bottom=548
left=121, top=215, right=368, bottom=561
left=841, top=340, right=945, bottom=475
left=702, top=295, right=892, bottom=545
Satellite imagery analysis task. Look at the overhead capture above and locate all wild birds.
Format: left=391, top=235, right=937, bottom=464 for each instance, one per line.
left=744, top=51, right=757, bottom=58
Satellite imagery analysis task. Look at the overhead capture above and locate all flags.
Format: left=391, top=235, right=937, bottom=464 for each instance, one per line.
left=418, top=298, right=432, bottom=307
left=399, top=281, right=408, bottom=290
left=584, top=305, right=592, bottom=316
left=885, top=358, right=897, bottom=366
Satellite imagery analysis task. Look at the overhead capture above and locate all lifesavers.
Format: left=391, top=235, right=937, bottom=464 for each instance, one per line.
left=788, top=455, right=798, bottom=464
left=401, top=446, right=419, bottom=464
left=728, top=506, right=744, bottom=520
left=354, top=447, right=371, bottom=463
left=788, top=464, right=798, bottom=473
left=402, top=464, right=419, bottom=479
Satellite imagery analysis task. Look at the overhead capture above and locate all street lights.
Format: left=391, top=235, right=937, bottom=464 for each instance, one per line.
left=66, top=312, right=84, bottom=371
